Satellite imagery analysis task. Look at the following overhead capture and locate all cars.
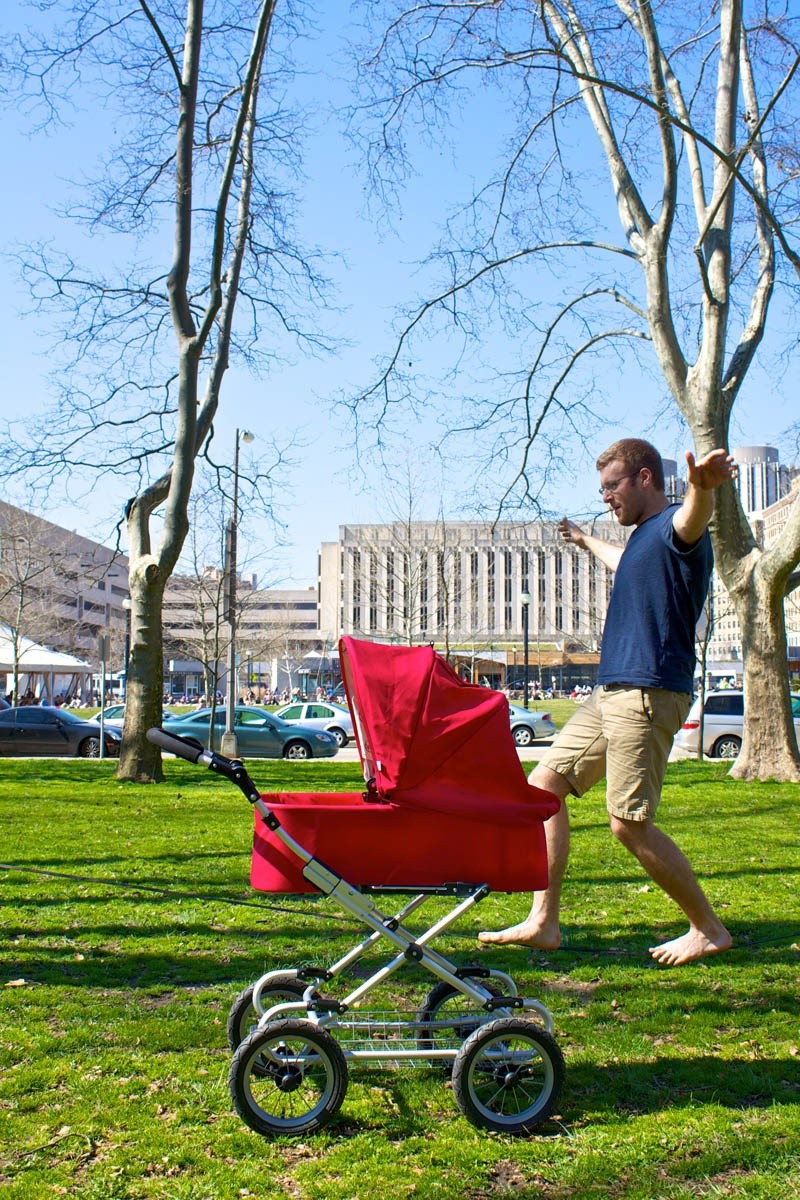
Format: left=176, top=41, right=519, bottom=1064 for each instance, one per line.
left=88, top=703, right=179, bottom=729
left=0, top=705, right=123, bottom=759
left=161, top=704, right=339, bottom=760
left=508, top=703, right=556, bottom=747
left=241, top=702, right=356, bottom=749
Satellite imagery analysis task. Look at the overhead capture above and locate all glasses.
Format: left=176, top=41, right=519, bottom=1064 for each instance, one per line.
left=599, top=469, right=641, bottom=495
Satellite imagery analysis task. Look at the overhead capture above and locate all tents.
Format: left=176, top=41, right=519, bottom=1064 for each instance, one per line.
left=0, top=620, right=97, bottom=715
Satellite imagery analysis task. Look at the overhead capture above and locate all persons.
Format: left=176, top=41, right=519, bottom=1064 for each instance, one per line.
left=476, top=438, right=739, bottom=970
left=482, top=677, right=749, bottom=704
left=4, top=686, right=348, bottom=713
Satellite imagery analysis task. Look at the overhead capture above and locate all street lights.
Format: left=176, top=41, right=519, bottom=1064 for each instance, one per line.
left=121, top=593, right=132, bottom=704
left=246, top=650, right=251, bottom=689
left=219, top=427, right=256, bottom=760
left=511, top=645, right=517, bottom=690
left=520, top=588, right=531, bottom=710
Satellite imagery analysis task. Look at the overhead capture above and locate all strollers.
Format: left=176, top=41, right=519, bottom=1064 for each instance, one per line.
left=143, top=634, right=565, bottom=1138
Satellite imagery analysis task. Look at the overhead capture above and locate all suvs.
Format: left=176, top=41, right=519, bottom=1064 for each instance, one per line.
left=673, top=688, right=800, bottom=759
